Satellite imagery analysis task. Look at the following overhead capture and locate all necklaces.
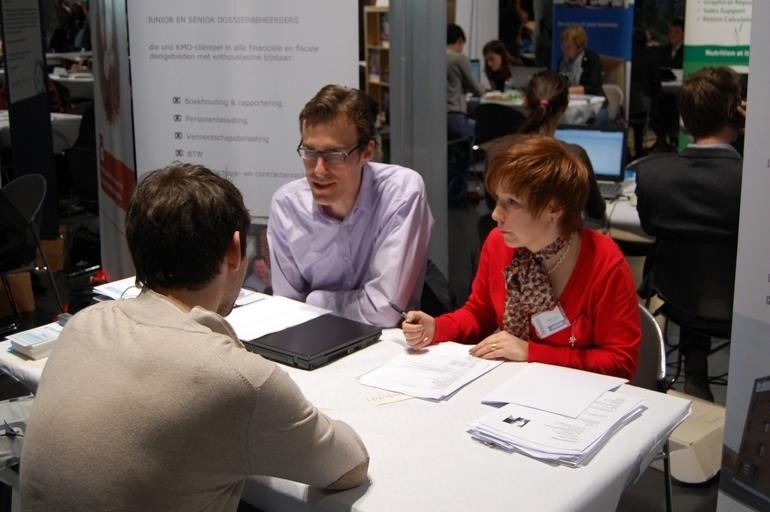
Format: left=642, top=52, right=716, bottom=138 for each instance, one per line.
left=545, top=240, right=571, bottom=276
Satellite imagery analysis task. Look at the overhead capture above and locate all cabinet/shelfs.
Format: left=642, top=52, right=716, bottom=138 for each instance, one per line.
left=362, top=2, right=390, bottom=129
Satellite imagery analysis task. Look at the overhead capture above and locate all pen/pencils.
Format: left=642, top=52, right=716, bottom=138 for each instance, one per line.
left=389, top=302, right=417, bottom=324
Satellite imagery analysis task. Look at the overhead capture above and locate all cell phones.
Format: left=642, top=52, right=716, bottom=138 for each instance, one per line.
left=726, top=106, right=746, bottom=128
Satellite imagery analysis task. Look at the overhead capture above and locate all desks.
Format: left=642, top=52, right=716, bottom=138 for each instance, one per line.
left=47, top=68, right=94, bottom=94
left=1, top=263, right=694, bottom=511
left=0, top=109, right=84, bottom=158
left=465, top=84, right=605, bottom=128
left=592, top=167, right=658, bottom=254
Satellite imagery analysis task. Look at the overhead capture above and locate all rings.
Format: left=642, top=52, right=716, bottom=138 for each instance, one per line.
left=490, top=342, right=498, bottom=349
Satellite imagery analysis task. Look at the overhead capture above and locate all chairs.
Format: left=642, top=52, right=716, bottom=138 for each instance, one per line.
left=599, top=83, right=624, bottom=127
left=8, top=96, right=55, bottom=160
left=1, top=171, right=51, bottom=224
left=1, top=208, right=68, bottom=330
left=632, top=292, right=669, bottom=386
left=630, top=146, right=743, bottom=396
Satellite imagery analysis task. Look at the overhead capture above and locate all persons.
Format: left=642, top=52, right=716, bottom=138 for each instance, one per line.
left=265, top=84, right=433, bottom=327
left=19, top=162, right=369, bottom=509
left=403, top=136, right=641, bottom=386
left=445, top=1, right=636, bottom=209
left=636, top=68, right=747, bottom=404
left=629, top=1, right=684, bottom=152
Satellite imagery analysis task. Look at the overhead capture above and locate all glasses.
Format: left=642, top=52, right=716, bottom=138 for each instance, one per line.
left=296, top=135, right=366, bottom=165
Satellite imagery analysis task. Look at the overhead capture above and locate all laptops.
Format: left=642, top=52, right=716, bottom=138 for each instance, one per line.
left=552, top=125, right=628, bottom=201
left=464, top=59, right=480, bottom=97
left=240, top=312, right=382, bottom=371
